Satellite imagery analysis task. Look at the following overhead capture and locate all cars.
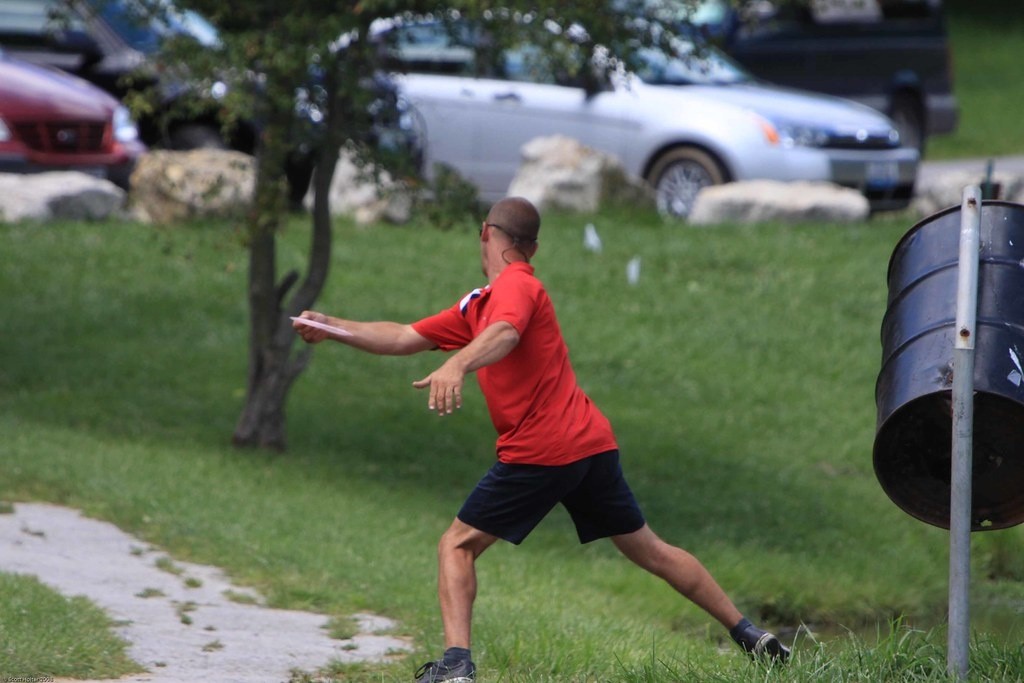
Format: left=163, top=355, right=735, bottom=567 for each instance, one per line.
left=0, top=0, right=274, bottom=190
left=634, top=0, right=959, bottom=163
left=295, top=0, right=922, bottom=228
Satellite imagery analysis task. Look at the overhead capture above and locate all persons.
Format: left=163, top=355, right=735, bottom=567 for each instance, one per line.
left=289, top=197, right=796, bottom=683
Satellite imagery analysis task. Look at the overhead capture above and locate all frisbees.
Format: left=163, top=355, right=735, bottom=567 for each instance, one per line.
left=291, top=315, right=355, bottom=339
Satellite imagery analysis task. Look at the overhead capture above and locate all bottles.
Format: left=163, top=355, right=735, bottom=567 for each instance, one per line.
left=584, top=223, right=602, bottom=255
left=626, top=253, right=642, bottom=289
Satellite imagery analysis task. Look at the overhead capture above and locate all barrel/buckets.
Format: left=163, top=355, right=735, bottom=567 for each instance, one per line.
left=872, top=202, right=1024, bottom=533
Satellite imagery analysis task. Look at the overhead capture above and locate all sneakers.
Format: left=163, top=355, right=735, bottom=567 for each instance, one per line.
left=739, top=625, right=790, bottom=663
left=415, top=659, right=476, bottom=683
